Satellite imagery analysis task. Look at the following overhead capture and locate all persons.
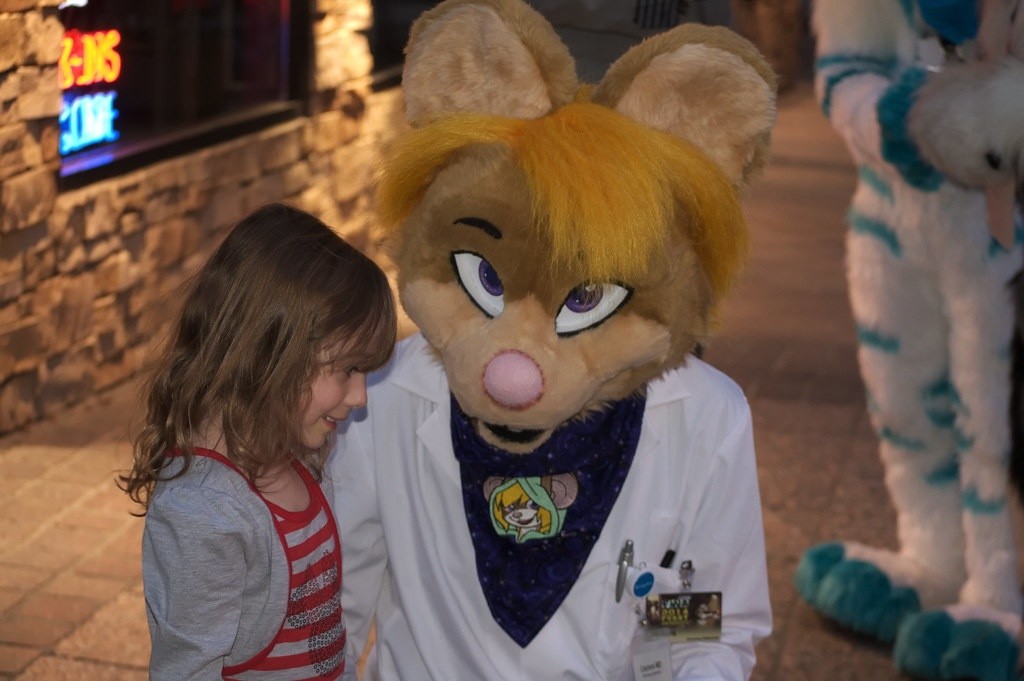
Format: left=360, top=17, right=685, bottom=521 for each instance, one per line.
left=110, top=202, right=397, bottom=681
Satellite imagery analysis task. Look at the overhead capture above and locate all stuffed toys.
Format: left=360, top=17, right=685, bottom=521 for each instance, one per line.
left=289, top=0, right=775, bottom=680
left=794, top=0, right=1024, bottom=680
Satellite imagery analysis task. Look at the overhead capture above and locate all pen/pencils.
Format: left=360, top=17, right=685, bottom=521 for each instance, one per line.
left=659, top=550, right=676, bottom=568
left=617, top=540, right=633, bottom=604
